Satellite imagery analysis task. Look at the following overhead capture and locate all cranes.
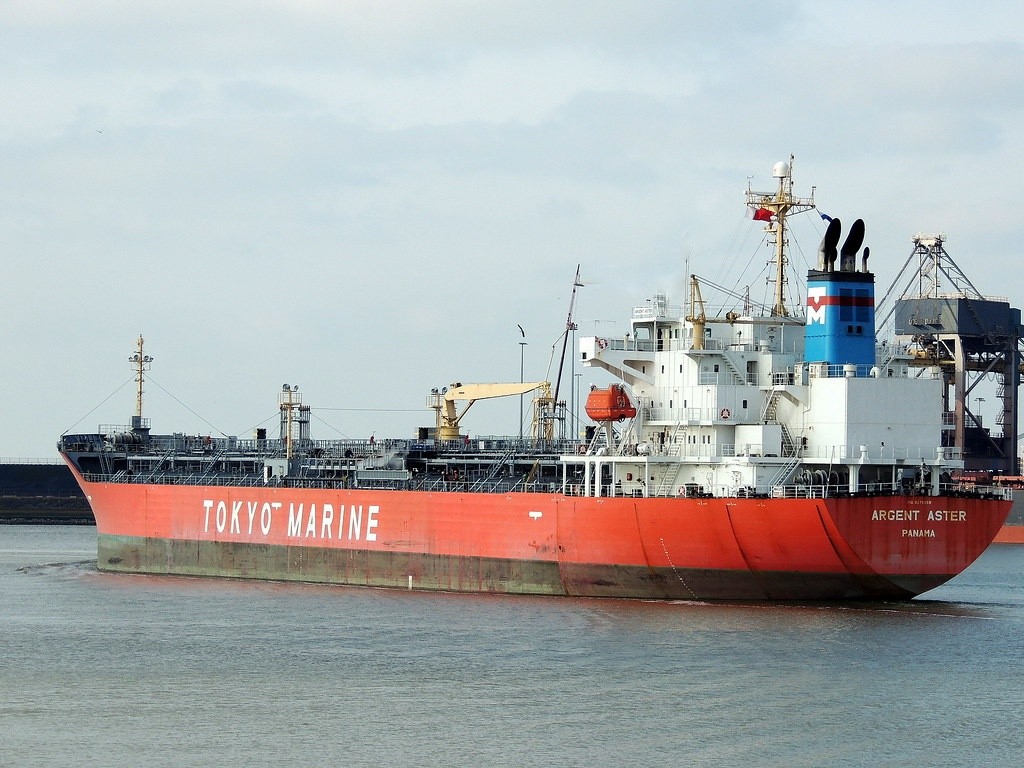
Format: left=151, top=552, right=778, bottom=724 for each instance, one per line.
left=439, top=379, right=554, bottom=449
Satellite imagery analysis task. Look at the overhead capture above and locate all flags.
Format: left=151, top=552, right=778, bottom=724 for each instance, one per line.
left=744, top=205, right=777, bottom=222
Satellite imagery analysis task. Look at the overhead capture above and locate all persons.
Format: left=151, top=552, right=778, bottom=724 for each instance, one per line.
left=370, top=434, right=375, bottom=446
left=441, top=470, right=459, bottom=481
left=343, top=447, right=354, bottom=467
left=465, top=434, right=470, bottom=450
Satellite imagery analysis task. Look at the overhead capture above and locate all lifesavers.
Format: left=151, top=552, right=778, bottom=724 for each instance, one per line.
left=598, top=338, right=608, bottom=348
left=579, top=445, right=587, bottom=455
left=721, top=408, right=731, bottom=419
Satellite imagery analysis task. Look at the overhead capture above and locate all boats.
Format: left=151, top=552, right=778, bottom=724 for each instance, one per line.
left=56, top=153, right=1017, bottom=603
